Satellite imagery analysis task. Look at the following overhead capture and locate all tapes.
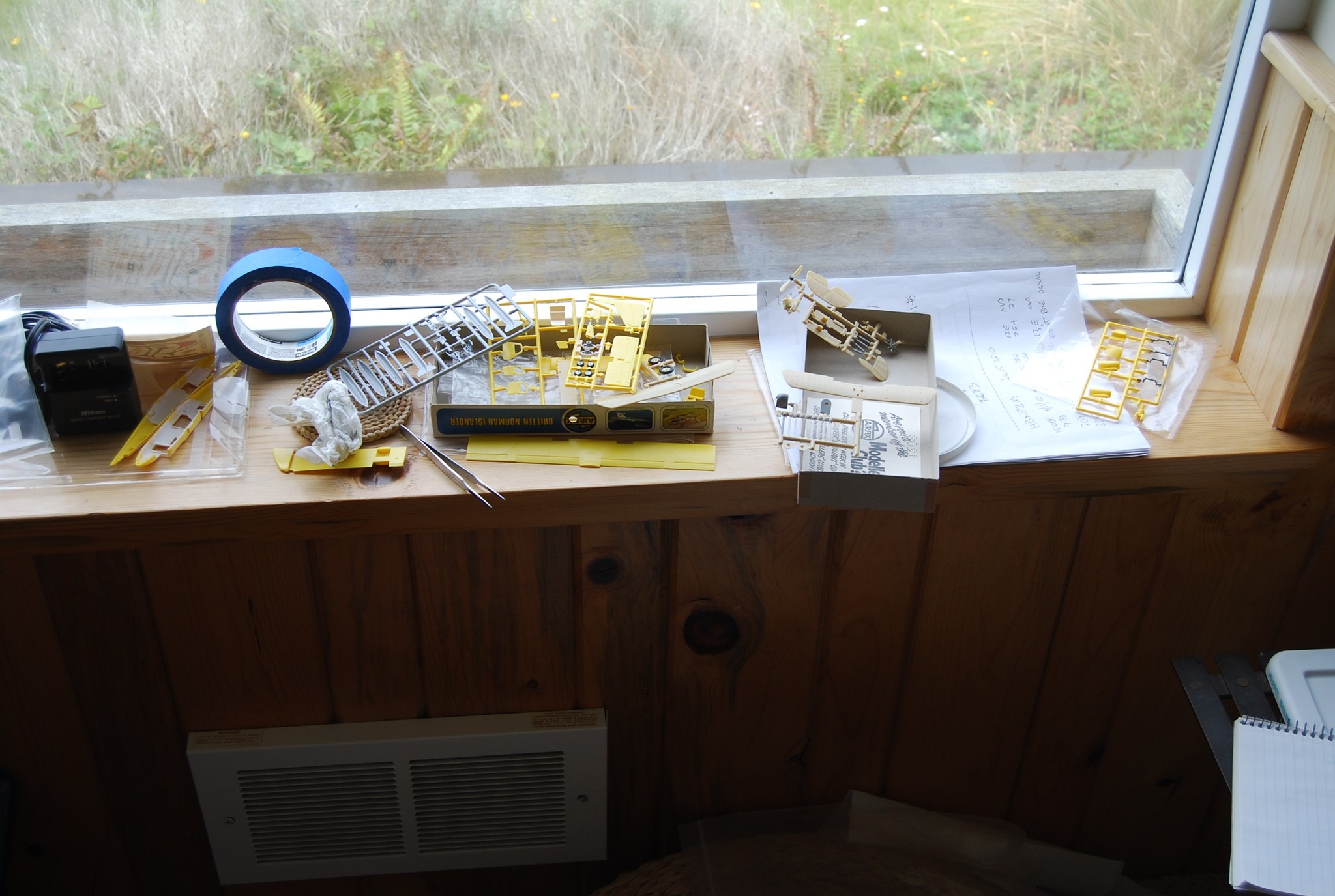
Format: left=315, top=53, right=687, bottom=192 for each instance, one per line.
left=215, top=247, right=349, bottom=375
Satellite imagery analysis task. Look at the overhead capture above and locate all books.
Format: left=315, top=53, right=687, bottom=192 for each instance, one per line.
left=1228, top=713, right=1335, bottom=896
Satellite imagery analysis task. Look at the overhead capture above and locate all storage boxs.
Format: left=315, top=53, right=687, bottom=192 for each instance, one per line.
left=798, top=307, right=940, bottom=512
left=430, top=323, right=715, bottom=437
